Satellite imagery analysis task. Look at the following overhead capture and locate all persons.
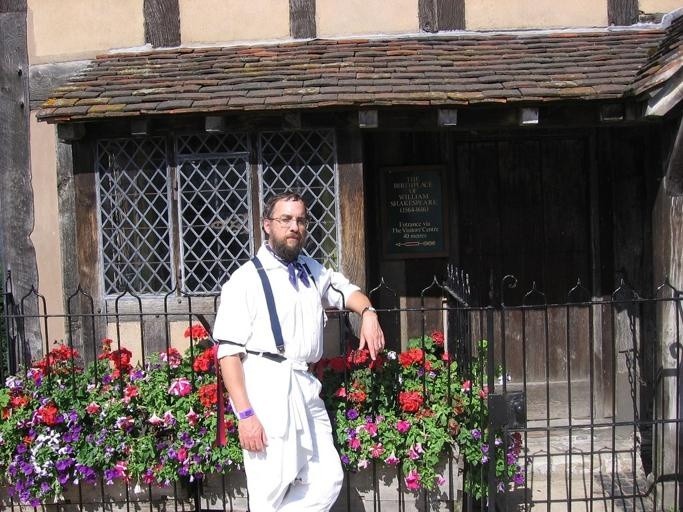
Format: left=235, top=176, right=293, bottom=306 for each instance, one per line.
left=211, top=192, right=385, bottom=512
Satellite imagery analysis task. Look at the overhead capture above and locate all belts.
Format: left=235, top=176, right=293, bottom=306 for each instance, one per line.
left=245, top=349, right=315, bottom=372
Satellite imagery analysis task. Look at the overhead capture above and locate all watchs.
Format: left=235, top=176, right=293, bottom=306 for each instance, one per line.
left=361, top=306, right=379, bottom=318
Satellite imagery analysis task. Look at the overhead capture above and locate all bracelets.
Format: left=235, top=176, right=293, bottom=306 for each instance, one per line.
left=239, top=408, right=254, bottom=420
